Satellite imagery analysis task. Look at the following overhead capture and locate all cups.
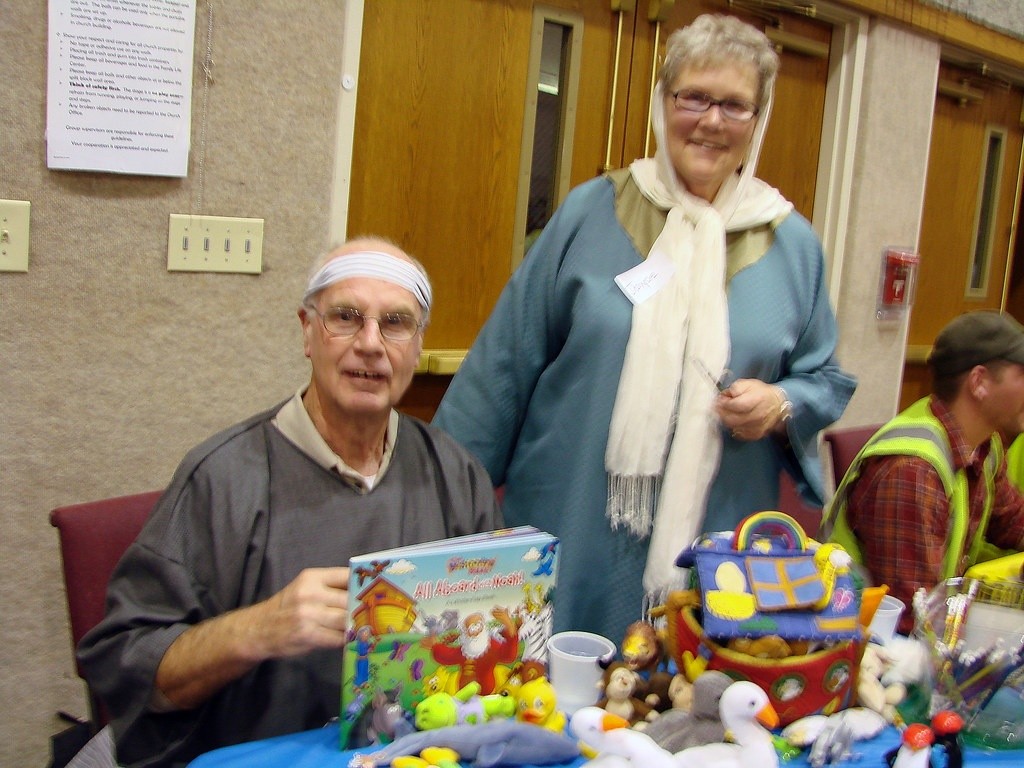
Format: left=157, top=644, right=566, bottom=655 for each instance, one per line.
left=868, top=595, right=904, bottom=642
left=546, top=631, right=615, bottom=716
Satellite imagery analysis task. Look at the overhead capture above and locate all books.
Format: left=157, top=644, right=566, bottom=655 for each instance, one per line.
left=339, top=525, right=561, bottom=751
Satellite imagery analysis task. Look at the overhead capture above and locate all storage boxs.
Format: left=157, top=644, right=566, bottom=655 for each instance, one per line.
left=677, top=604, right=866, bottom=726
left=961, top=553, right=1024, bottom=656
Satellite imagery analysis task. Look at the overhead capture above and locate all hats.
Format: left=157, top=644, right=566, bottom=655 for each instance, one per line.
left=926, top=309, right=1024, bottom=373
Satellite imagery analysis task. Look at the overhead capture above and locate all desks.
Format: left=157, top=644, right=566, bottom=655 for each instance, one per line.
left=185, top=660, right=1024, bottom=767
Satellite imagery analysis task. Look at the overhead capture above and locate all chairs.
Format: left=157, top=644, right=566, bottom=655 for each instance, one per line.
left=824, top=424, right=884, bottom=493
left=49, top=493, right=167, bottom=679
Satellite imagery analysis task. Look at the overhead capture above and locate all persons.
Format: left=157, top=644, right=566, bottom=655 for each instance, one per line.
left=75, top=239, right=505, bottom=768
left=428, top=13, right=859, bottom=677
left=817, top=310, right=1024, bottom=642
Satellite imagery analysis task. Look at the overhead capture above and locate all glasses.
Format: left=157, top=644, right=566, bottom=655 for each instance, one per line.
left=309, top=304, right=424, bottom=341
left=665, top=88, right=761, bottom=123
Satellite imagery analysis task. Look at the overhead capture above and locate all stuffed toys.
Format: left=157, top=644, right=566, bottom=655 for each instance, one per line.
left=348, top=584, right=965, bottom=768
